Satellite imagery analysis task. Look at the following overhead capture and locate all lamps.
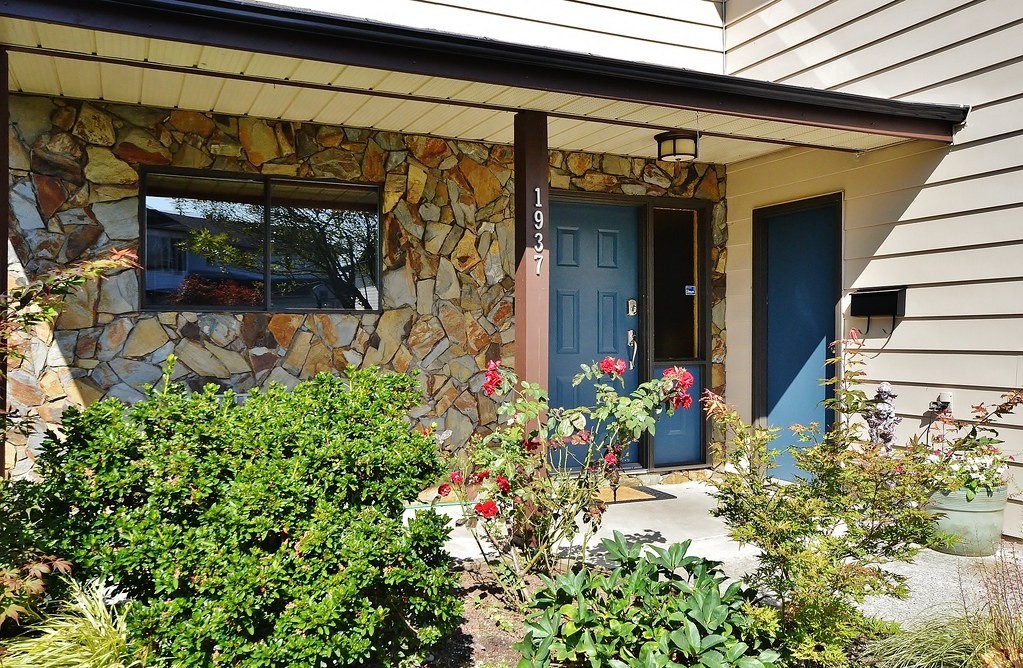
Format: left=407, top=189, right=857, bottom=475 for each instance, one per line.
left=654, top=130, right=702, bottom=162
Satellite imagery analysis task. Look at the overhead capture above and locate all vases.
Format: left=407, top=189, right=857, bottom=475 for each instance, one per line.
left=922, top=480, right=1007, bottom=557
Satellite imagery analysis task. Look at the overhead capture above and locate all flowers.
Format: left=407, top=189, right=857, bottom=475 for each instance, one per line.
left=901, top=421, right=1016, bottom=500
